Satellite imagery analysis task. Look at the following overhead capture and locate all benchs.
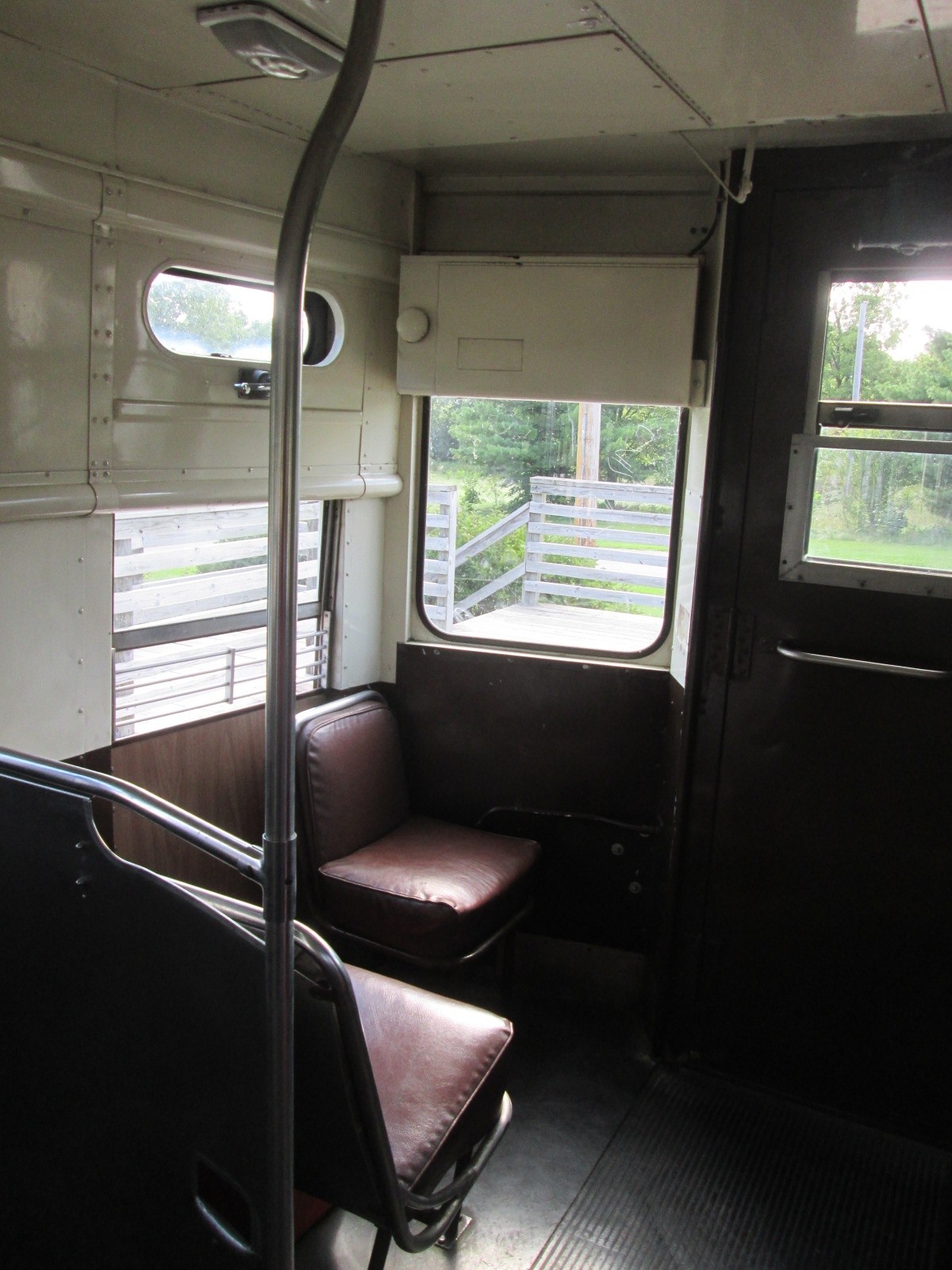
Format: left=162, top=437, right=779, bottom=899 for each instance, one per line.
left=0, top=752, right=518, bottom=1270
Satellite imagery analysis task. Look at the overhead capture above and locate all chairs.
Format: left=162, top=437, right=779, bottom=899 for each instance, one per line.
left=287, top=684, right=539, bottom=1005
